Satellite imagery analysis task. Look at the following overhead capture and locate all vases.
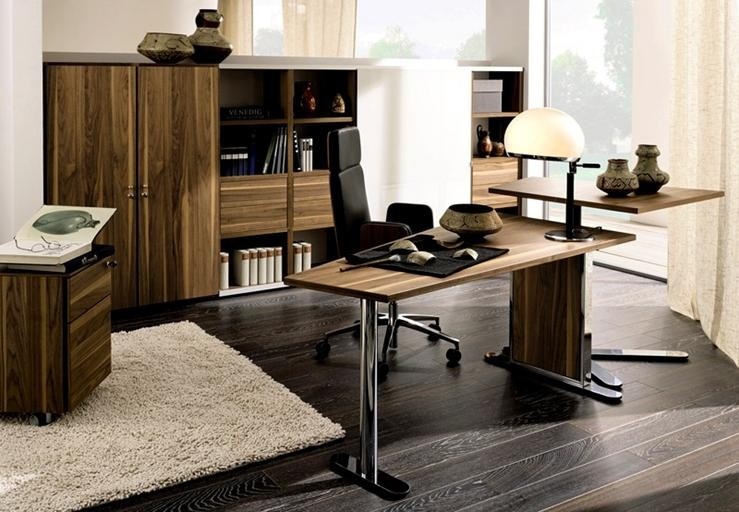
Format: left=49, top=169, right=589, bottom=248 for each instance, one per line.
left=187, top=8, right=233, bottom=65
left=299, top=81, right=317, bottom=118
left=595, top=158, right=641, bottom=197
left=632, top=143, right=670, bottom=194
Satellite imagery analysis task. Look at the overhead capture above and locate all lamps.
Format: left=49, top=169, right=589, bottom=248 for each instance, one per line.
left=504, top=106, right=604, bottom=243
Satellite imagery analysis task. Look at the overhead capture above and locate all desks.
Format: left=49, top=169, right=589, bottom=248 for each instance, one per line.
left=282, top=174, right=725, bottom=503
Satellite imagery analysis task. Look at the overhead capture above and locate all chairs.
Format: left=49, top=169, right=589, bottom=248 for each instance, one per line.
left=315, top=125, right=462, bottom=383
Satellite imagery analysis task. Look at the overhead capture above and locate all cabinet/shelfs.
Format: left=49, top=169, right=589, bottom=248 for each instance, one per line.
left=469, top=65, right=525, bottom=219
left=0, top=245, right=118, bottom=427
left=43, top=62, right=221, bottom=311
left=219, top=68, right=357, bottom=299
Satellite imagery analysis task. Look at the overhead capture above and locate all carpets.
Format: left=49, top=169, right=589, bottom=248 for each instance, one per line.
left=0, top=319, right=350, bottom=512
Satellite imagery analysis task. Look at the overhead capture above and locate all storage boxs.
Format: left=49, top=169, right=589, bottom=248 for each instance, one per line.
left=471, top=79, right=504, bottom=113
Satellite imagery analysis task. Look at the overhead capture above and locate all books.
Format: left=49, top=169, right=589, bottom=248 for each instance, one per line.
left=219, top=105, right=315, bottom=178
left=217, top=240, right=313, bottom=291
left=0, top=205, right=119, bottom=267
